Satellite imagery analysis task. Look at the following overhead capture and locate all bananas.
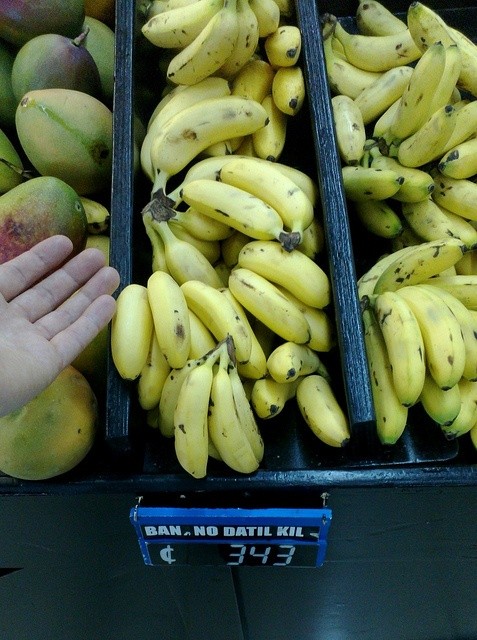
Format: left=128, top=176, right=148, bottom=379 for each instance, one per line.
left=111, top=0, right=350, bottom=480
left=322, top=0, right=477, bottom=449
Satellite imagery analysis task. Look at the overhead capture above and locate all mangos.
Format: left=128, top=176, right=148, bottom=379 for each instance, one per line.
left=0, top=0, right=115, bottom=481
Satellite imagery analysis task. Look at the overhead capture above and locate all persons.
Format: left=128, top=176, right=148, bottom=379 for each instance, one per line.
left=2, top=230, right=121, bottom=411
